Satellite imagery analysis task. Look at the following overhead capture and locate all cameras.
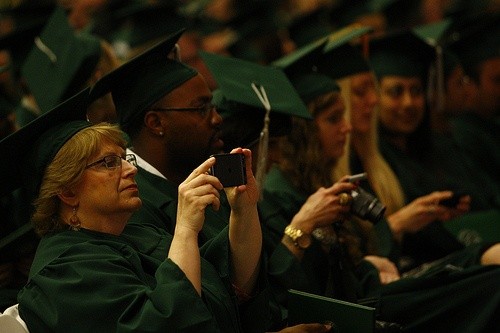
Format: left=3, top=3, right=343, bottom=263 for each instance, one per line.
left=336, top=172, right=387, bottom=226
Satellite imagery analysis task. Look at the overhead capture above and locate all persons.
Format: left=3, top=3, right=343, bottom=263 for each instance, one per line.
left=0, top=0, right=500, bottom=333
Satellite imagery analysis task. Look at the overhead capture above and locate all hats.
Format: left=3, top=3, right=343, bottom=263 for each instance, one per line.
left=369, top=18, right=458, bottom=114
left=271, top=36, right=341, bottom=107
left=1, top=87, right=93, bottom=193
left=325, top=27, right=372, bottom=81
left=198, top=50, right=313, bottom=202
left=93, top=26, right=197, bottom=135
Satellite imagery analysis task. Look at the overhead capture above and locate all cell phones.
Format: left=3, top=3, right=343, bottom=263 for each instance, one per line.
left=436, top=196, right=460, bottom=209
left=209, top=152, right=246, bottom=187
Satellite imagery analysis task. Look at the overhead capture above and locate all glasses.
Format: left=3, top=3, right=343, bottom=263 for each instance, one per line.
left=86, top=154, right=137, bottom=170
left=156, top=96, right=221, bottom=115
